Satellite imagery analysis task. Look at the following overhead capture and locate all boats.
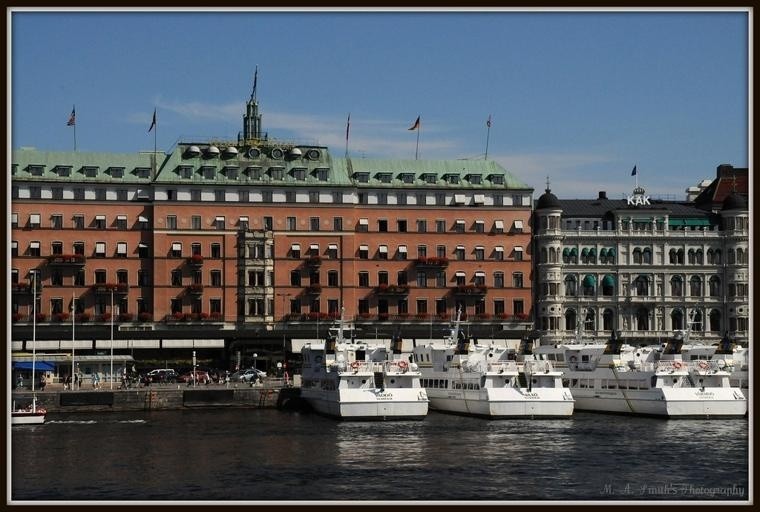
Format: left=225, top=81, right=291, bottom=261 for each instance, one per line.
left=10, top=399, right=50, bottom=427
left=408, top=307, right=749, bottom=422
left=298, top=308, right=432, bottom=426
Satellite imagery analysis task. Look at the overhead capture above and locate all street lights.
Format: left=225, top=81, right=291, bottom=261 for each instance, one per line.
left=253, top=353, right=259, bottom=372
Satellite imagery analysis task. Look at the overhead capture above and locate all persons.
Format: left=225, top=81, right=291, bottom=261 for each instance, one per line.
left=16, top=368, right=291, bottom=392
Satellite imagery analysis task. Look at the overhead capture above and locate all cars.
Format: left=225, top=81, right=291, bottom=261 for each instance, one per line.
left=136, top=362, right=269, bottom=386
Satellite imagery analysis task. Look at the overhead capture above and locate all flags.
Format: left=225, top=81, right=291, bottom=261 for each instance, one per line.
left=407, top=114, right=420, bottom=131
left=64, top=106, right=76, bottom=127
left=344, top=113, right=350, bottom=141
left=630, top=165, right=637, bottom=177
left=147, top=111, right=156, bottom=133
